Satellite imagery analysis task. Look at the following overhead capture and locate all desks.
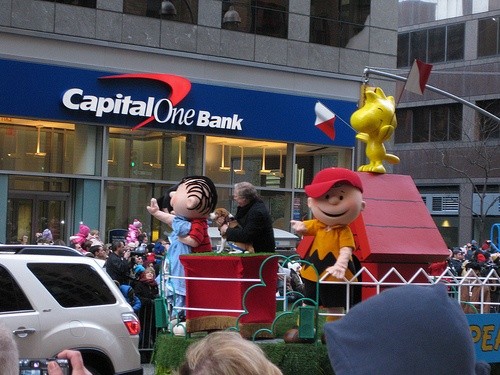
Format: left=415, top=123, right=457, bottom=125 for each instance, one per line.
left=179, top=252, right=278, bottom=340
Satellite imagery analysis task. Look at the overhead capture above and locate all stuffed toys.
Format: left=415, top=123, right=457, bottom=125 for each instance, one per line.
left=145, top=175, right=219, bottom=336
left=290, top=167, right=366, bottom=334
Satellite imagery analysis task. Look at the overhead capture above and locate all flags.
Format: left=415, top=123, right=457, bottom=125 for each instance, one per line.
left=404, top=58, right=434, bottom=97
left=313, top=99, right=338, bottom=141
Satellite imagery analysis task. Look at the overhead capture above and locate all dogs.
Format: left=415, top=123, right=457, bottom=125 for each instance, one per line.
left=209, top=208, right=255, bottom=254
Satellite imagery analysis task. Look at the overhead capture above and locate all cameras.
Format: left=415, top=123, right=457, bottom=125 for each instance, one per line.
left=18, top=358, right=72, bottom=375
left=124, top=249, right=146, bottom=256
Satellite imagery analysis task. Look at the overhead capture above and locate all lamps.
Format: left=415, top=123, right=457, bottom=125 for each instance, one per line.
left=8, top=130, right=21, bottom=159
left=149, top=140, right=162, bottom=169
left=34, top=125, right=47, bottom=157
left=176, top=140, right=185, bottom=169
left=108, top=137, right=118, bottom=166
left=219, top=145, right=230, bottom=173
left=233, top=146, right=246, bottom=175
left=274, top=150, right=284, bottom=178
left=222, top=5, right=241, bottom=26
left=158, top=0, right=177, bottom=16
left=259, top=146, right=271, bottom=176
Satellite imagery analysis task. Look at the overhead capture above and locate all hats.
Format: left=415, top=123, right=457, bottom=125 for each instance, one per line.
left=305, top=166, right=363, bottom=198
left=133, top=219, right=142, bottom=227
left=78, top=224, right=90, bottom=237
left=472, top=239, right=477, bottom=244
left=453, top=249, right=464, bottom=255
left=154, top=242, right=165, bottom=253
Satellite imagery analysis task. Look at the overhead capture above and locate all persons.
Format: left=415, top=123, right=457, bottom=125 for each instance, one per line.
left=430, top=240, right=500, bottom=313
left=0, top=325, right=284, bottom=375
left=219, top=181, right=308, bottom=311
left=323, top=282, right=491, bottom=375
left=16, top=219, right=171, bottom=347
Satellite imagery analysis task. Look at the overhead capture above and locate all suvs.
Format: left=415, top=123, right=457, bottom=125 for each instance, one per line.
left=0, top=243, right=144, bottom=375
